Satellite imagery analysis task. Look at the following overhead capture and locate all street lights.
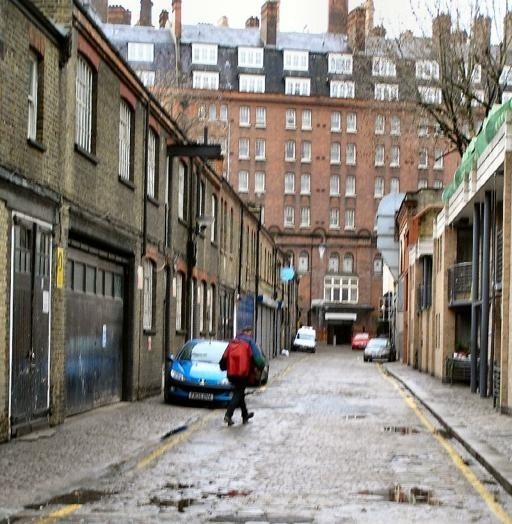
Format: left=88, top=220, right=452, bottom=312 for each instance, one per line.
left=185, top=214, right=214, bottom=340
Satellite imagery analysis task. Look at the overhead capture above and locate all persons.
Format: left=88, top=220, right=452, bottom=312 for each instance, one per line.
left=219, top=324, right=267, bottom=425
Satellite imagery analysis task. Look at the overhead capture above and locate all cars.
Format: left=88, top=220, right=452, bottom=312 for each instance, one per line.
left=162, top=338, right=237, bottom=405
left=363, top=337, right=395, bottom=362
left=351, top=332, right=370, bottom=350
left=293, top=326, right=317, bottom=353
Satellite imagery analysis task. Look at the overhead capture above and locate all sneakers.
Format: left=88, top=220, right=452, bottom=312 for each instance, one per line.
left=242, top=412, right=254, bottom=423
left=224, top=414, right=235, bottom=425
